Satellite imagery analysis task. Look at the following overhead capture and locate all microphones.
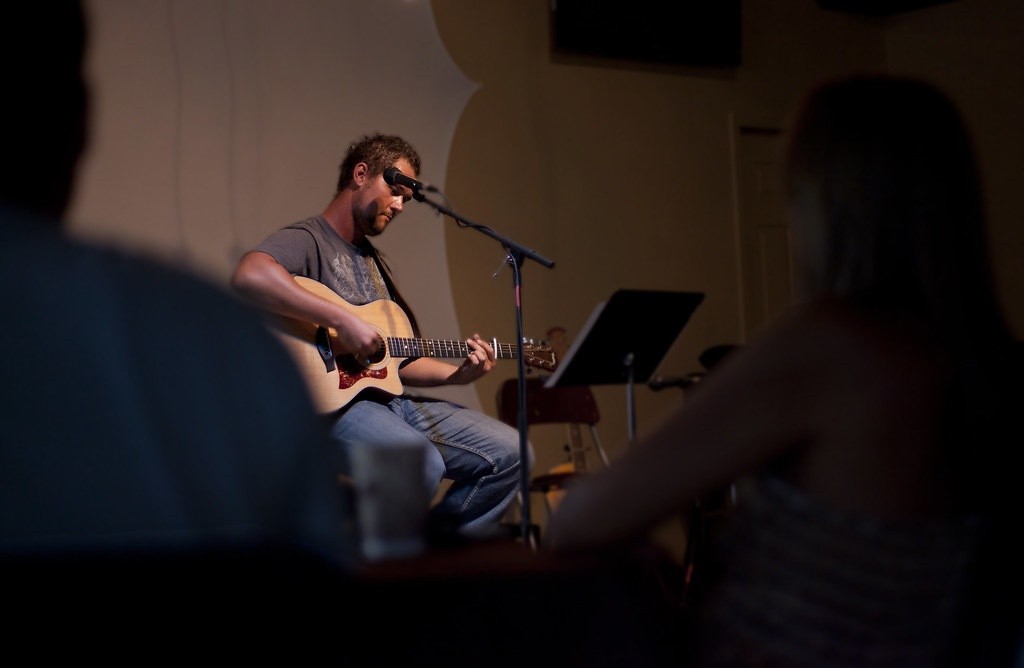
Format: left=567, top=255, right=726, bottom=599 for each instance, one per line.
left=383, top=167, right=439, bottom=193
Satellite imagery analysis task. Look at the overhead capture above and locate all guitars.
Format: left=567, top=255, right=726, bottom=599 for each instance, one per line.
left=266, top=274, right=558, bottom=416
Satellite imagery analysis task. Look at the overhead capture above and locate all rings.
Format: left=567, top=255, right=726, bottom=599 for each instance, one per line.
left=355, top=352, right=359, bottom=360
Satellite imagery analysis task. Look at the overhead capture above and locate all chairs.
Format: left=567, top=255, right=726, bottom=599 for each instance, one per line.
left=497, top=377, right=610, bottom=560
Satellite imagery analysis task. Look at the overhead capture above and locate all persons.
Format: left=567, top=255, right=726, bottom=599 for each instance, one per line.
left=231, top=135, right=534, bottom=552
left=0, top=1, right=340, bottom=558
left=542, top=68, right=1024, bottom=668
